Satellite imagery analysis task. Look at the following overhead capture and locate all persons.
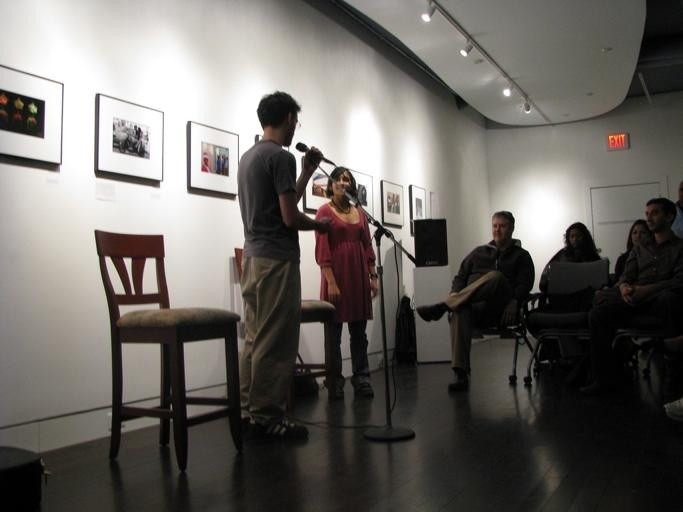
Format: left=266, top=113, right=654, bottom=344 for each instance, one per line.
left=540, top=182, right=682, bottom=420
left=418, top=211, right=534, bottom=394
left=238, top=92, right=336, bottom=445
left=316, top=166, right=381, bottom=403
left=388, top=195, right=400, bottom=214
left=204, top=152, right=226, bottom=173
left=132, top=126, right=143, bottom=145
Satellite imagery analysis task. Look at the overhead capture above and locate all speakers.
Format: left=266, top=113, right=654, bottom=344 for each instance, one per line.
left=414, top=218, right=448, bottom=267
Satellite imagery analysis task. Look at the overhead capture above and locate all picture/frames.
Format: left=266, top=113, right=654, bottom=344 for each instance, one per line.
left=0, top=63, right=63, bottom=170
left=187, top=122, right=238, bottom=201
left=93, top=92, right=164, bottom=186
left=255, top=134, right=440, bottom=237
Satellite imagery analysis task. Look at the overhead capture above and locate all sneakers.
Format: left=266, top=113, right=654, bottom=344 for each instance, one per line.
left=249, top=417, right=309, bottom=444
left=448, top=375, right=471, bottom=391
left=354, top=382, right=374, bottom=400
left=328, top=385, right=344, bottom=401
left=416, top=304, right=443, bottom=322
left=663, top=398, right=683, bottom=421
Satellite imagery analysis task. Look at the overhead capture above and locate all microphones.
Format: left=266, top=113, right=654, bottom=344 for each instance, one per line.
left=296, top=142, right=335, bottom=166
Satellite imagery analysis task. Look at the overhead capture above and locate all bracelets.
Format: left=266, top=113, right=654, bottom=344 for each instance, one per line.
left=368, top=272, right=377, bottom=279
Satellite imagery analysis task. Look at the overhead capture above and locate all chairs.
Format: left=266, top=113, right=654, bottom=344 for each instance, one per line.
left=93, top=229, right=243, bottom=470
left=448, top=279, right=682, bottom=396
left=235, top=247, right=343, bottom=423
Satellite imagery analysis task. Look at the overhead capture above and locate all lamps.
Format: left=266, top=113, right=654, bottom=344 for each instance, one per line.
left=419, top=0, right=548, bottom=123
